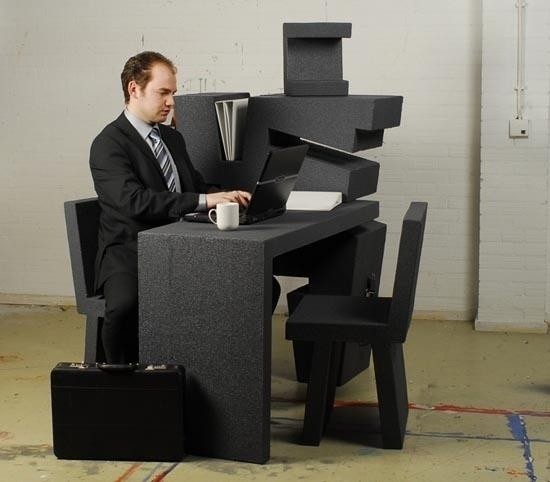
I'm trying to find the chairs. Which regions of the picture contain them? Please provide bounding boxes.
[63,197,110,360]
[284,202,430,450]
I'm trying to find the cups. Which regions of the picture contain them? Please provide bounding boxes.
[208,202,240,231]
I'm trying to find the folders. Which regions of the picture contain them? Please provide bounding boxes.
[214,98,248,162]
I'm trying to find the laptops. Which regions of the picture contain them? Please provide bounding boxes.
[183,144,309,225]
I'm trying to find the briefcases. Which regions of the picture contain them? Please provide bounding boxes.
[50,361,188,463]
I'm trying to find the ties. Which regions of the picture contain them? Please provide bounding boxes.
[148,127,177,193]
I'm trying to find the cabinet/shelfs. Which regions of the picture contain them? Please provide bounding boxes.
[169,22,405,200]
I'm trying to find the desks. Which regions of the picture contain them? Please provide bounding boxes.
[139,201,406,466]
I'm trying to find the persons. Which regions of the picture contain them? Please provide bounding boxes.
[85,48,253,366]
